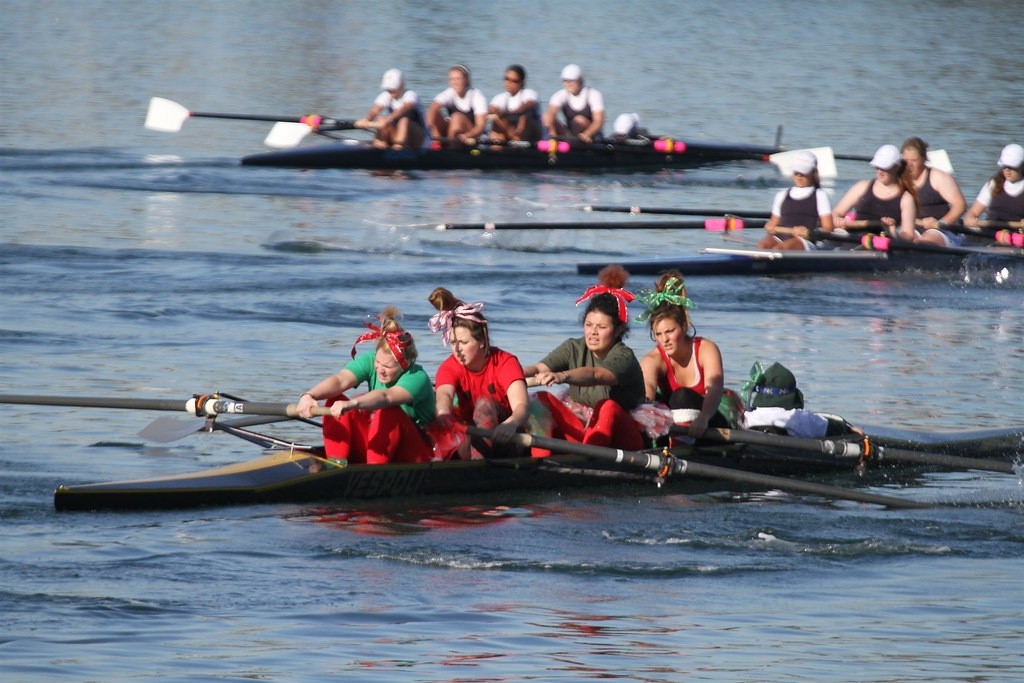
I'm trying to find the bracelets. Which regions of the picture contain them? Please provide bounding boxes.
[937,219,945,227]
[560,371,568,383]
[299,392,314,398]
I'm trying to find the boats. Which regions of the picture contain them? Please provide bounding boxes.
[576,246,1024,274]
[241,142,780,171]
[53,423,1024,511]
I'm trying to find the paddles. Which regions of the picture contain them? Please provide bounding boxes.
[957,219,1023,231]
[915,218,1024,248]
[513,195,857,221]
[430,135,571,157]
[526,377,550,387]
[434,214,894,233]
[264,120,359,149]
[141,94,383,139]
[0,393,345,419]
[752,144,954,176]
[763,224,1024,265]
[137,415,290,444]
[444,422,923,509]
[667,425,1019,474]
[557,137,688,163]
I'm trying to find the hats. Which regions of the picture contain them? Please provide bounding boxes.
[740,361,804,411]
[870,145,903,170]
[997,144,1024,167]
[791,150,817,175]
[614,113,640,135]
[379,69,404,89]
[560,64,581,81]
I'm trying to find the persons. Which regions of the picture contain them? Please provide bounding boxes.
[486,64,543,149]
[757,151,834,250]
[965,143,1024,245]
[428,288,530,460]
[635,270,735,449]
[719,362,866,438]
[296,306,436,465]
[427,65,488,149]
[540,65,605,143]
[831,139,968,249]
[524,265,652,459]
[355,69,426,147]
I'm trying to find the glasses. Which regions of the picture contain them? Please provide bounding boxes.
[504,75,522,83]
